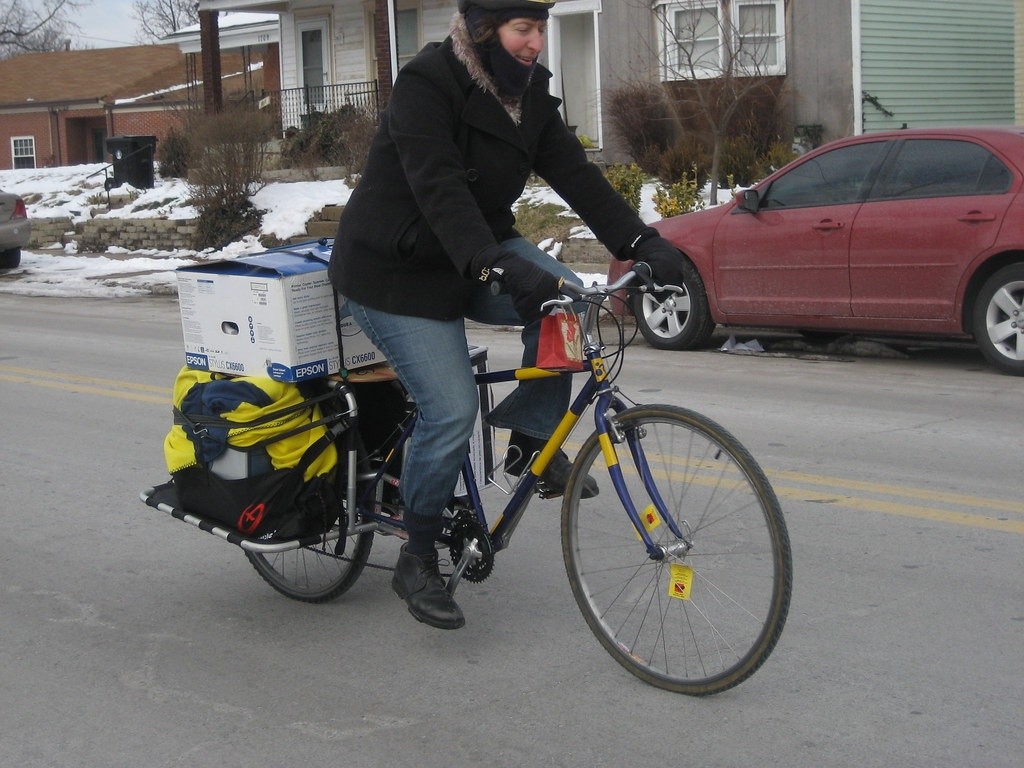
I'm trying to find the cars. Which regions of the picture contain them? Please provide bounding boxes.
[0,189,32,267]
[607,125,1023,375]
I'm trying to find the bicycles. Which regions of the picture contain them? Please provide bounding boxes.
[139,260,797,696]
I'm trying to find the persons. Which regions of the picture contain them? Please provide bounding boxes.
[326,0,683,629]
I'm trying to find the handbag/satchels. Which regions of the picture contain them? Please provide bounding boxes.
[536,296,584,372]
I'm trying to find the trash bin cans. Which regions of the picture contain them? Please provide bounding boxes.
[105,135,156,192]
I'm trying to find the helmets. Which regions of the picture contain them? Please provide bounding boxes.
[457,0,556,14]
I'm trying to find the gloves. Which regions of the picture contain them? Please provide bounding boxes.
[624,227,684,303]
[471,243,558,322]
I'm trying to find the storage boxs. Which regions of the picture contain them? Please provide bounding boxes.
[174,235,387,383]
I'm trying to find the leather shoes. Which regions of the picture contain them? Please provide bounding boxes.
[392,542,465,629]
[504,430,599,499]
